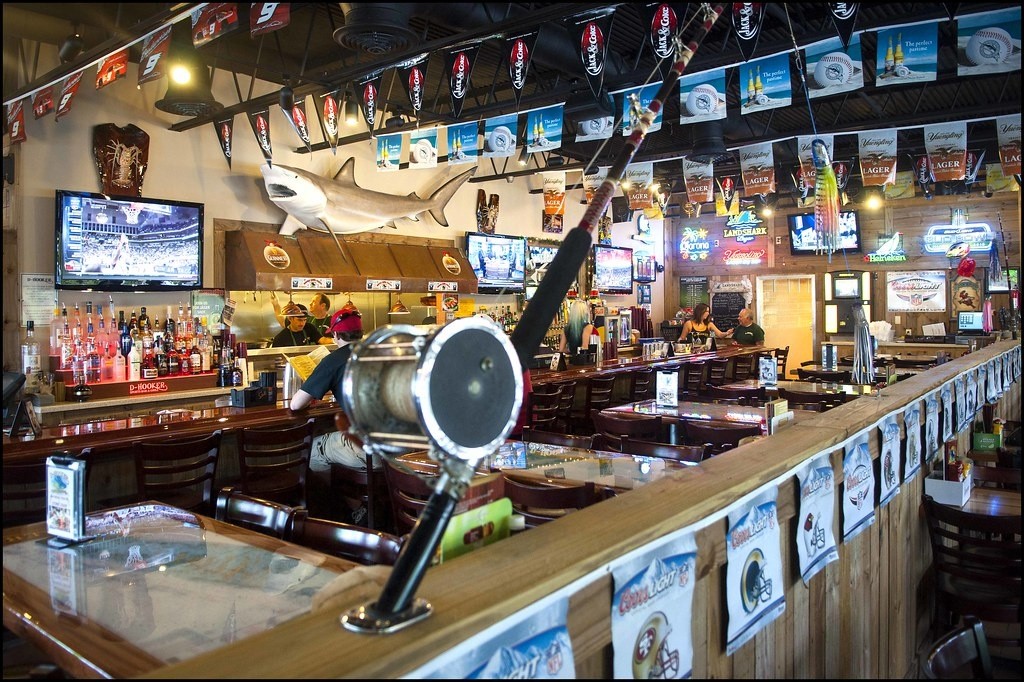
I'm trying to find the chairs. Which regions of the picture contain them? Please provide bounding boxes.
[0,345,1024,682]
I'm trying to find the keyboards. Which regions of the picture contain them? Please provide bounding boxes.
[963,332,983,336]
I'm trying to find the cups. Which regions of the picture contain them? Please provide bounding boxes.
[642,341,665,361]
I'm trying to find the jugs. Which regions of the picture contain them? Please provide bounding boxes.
[282,362,302,400]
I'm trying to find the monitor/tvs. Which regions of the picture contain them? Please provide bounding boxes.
[593,242,634,295]
[464,232,525,295]
[787,209,862,256]
[54,190,203,293]
[957,310,984,333]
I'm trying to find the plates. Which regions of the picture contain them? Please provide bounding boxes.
[442,297,458,311]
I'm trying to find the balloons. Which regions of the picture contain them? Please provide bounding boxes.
[958,257,975,278]
[946,242,970,259]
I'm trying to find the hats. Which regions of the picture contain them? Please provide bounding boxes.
[291,304,312,318]
[325,308,363,334]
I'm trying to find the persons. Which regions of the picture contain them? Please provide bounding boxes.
[560,300,594,354]
[270,292,333,338]
[290,309,414,527]
[272,304,335,347]
[731,309,764,346]
[676,303,734,351]
[111,234,130,275]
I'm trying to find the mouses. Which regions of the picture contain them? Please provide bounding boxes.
[984,333,990,336]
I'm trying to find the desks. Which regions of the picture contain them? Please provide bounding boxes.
[968,444,1024,463]
[949,488,1024,566]
[720,380,875,404]
[846,354,937,365]
[599,398,815,437]
[789,366,924,380]
[400,439,701,504]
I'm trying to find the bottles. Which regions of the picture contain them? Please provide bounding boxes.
[472,297,571,350]
[709,330,715,338]
[50,300,243,388]
[20,320,44,392]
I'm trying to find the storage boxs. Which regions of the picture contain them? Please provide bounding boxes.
[925,470,970,508]
[972,431,1002,451]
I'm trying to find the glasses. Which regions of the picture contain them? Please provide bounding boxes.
[331,310,362,330]
